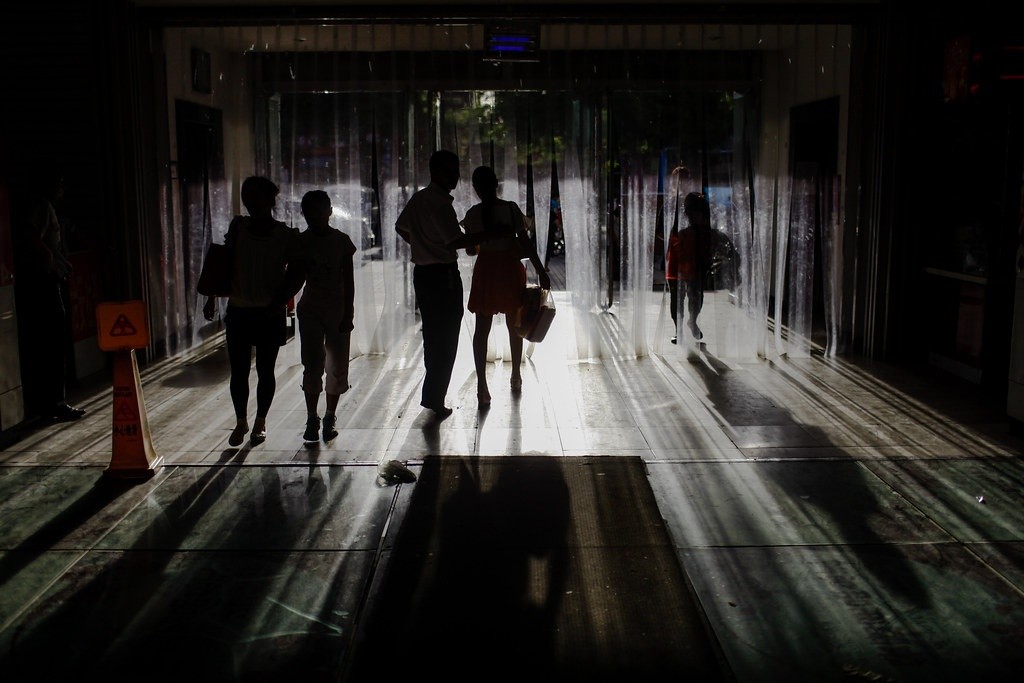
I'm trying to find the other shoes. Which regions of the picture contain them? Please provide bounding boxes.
[510,374,522,391]
[421,398,452,415]
[477,389,491,405]
[303,417,321,441]
[229,423,249,446]
[687,321,703,339]
[671,334,682,344]
[250,420,266,442]
[322,416,338,442]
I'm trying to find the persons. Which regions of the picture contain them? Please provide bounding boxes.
[289,187,355,441]
[203,176,298,446]
[666,165,706,343]
[458,165,552,400]
[24,168,86,422]
[395,149,471,415]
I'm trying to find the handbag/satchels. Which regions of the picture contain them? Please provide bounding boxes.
[197,243,230,297]
[516,286,555,342]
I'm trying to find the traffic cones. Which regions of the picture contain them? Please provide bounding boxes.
[93,298,166,479]
